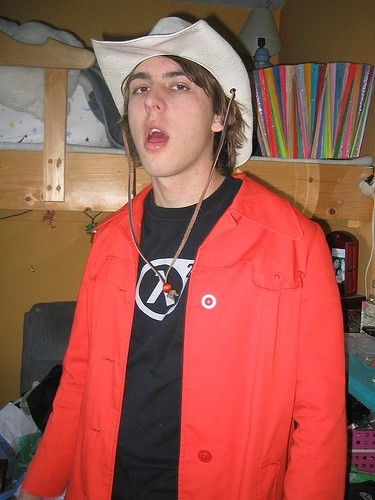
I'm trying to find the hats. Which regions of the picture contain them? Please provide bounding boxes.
[90,16,254,168]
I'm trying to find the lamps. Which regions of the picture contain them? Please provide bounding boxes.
[234,7,282,70]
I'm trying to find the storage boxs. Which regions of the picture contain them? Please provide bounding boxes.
[348,428,375,472]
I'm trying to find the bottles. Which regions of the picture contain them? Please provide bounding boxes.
[254,47,273,70]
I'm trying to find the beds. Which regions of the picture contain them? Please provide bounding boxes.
[1,31,374,229]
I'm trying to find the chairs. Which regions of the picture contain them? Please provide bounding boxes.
[0,299,78,493]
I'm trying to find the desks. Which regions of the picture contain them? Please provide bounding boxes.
[345,351,374,415]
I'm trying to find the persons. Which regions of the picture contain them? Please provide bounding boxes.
[19,14,345,500]
